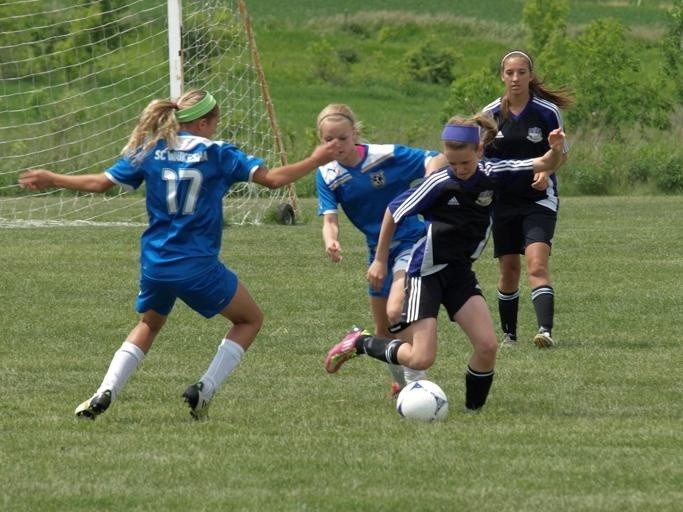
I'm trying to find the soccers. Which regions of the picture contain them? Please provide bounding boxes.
[396,379,448,422]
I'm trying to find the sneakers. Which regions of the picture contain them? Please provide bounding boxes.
[532,326,555,349]
[182,381,212,423]
[387,382,401,405]
[74,390,111,421]
[323,324,373,373]
[499,332,518,349]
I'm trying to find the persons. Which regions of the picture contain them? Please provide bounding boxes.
[16,86,342,420]
[322,110,569,418]
[313,101,450,400]
[472,50,576,351]
